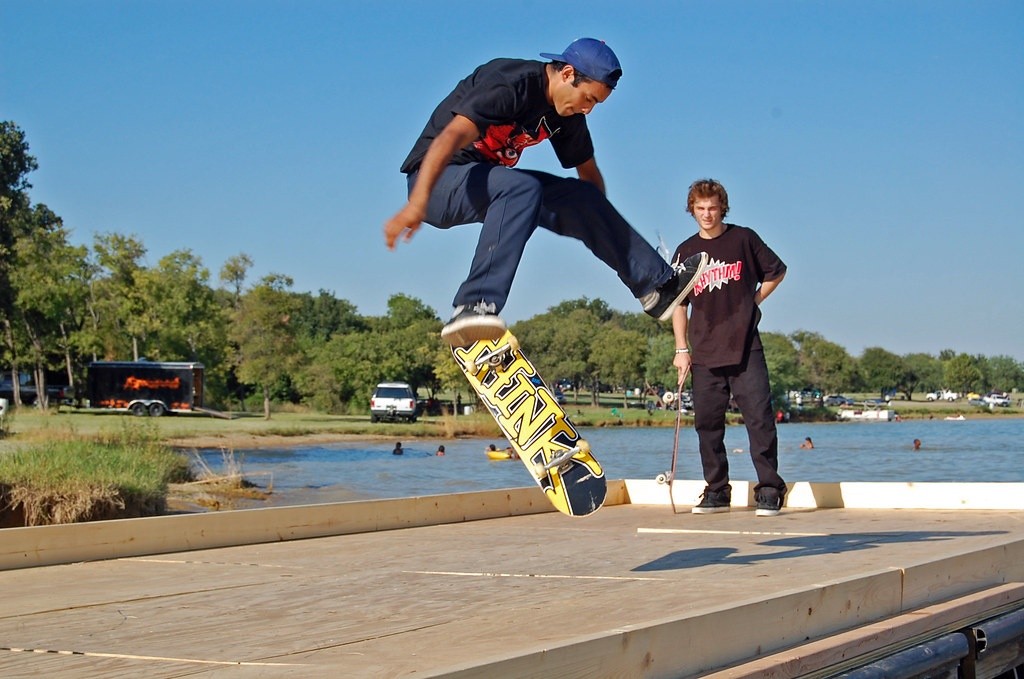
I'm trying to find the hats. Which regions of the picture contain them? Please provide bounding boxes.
[540,38,623,90]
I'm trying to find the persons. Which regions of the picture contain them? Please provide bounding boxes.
[436,445,445,456]
[669,180,787,516]
[912,439,921,450]
[800,437,813,449]
[393,442,403,454]
[386,37,706,347]
[485,444,520,460]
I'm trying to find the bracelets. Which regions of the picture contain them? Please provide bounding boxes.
[675,349,690,353]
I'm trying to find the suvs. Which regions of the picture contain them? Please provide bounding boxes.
[370,381,417,423]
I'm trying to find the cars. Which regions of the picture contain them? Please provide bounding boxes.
[555,390,566,403]
[826,396,853,407]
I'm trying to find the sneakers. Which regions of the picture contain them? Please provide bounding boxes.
[645,252,709,321]
[439,307,506,345]
[690,492,731,514]
[755,486,783,516]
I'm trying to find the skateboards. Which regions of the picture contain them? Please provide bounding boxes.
[449,327,607,516]
[655,360,690,514]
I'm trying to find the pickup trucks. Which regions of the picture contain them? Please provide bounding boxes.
[983,394,1009,406]
[927,390,958,402]
[0,381,63,406]
